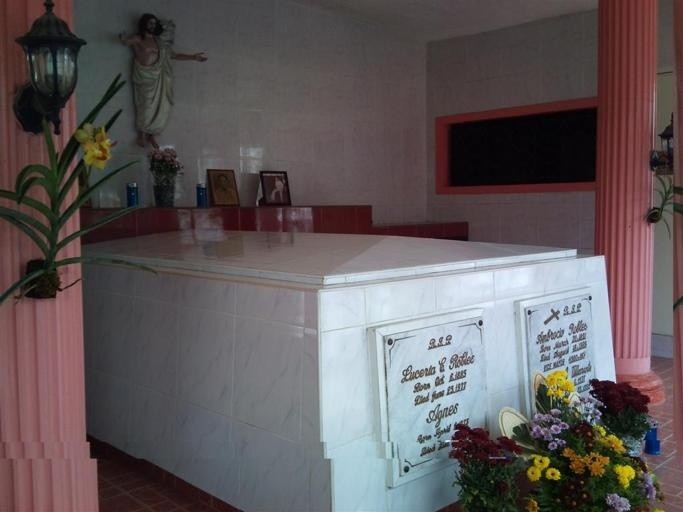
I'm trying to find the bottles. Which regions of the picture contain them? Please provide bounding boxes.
[196,183,208,208]
[126,182,138,208]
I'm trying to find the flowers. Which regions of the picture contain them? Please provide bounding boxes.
[447,369,668,512]
[0,71,163,298]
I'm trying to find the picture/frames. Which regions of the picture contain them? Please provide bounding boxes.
[206,169,240,207]
[255,170,292,207]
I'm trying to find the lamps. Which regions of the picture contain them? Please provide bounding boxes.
[13,1,88,136]
[649,112,675,175]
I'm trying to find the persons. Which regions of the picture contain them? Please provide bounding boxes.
[120,14,208,149]
[216,174,237,202]
[271,177,284,203]
